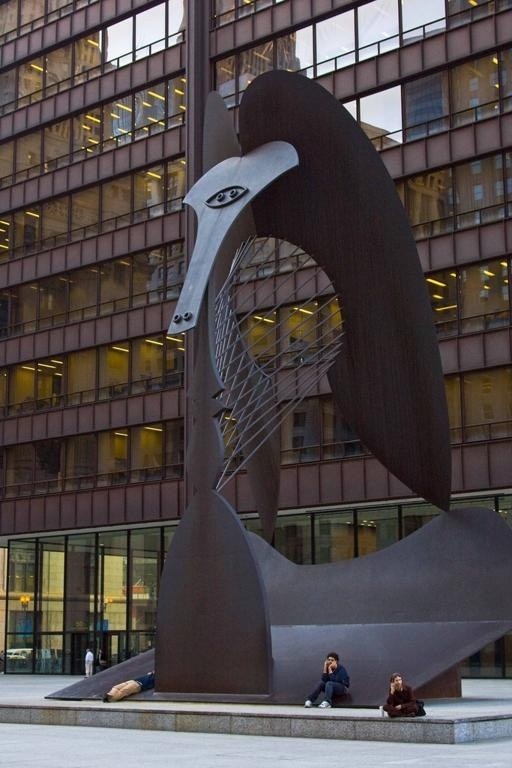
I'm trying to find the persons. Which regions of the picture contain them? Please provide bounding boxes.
[305,652,351,708]
[103,671,155,703]
[96,647,106,672]
[383,673,418,719]
[84,648,95,679]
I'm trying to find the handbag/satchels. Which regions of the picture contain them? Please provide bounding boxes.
[417,699,426,716]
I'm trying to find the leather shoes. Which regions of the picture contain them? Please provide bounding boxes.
[103,690,108,703]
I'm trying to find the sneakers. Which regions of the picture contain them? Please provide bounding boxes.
[304,700,313,708]
[318,701,332,708]
